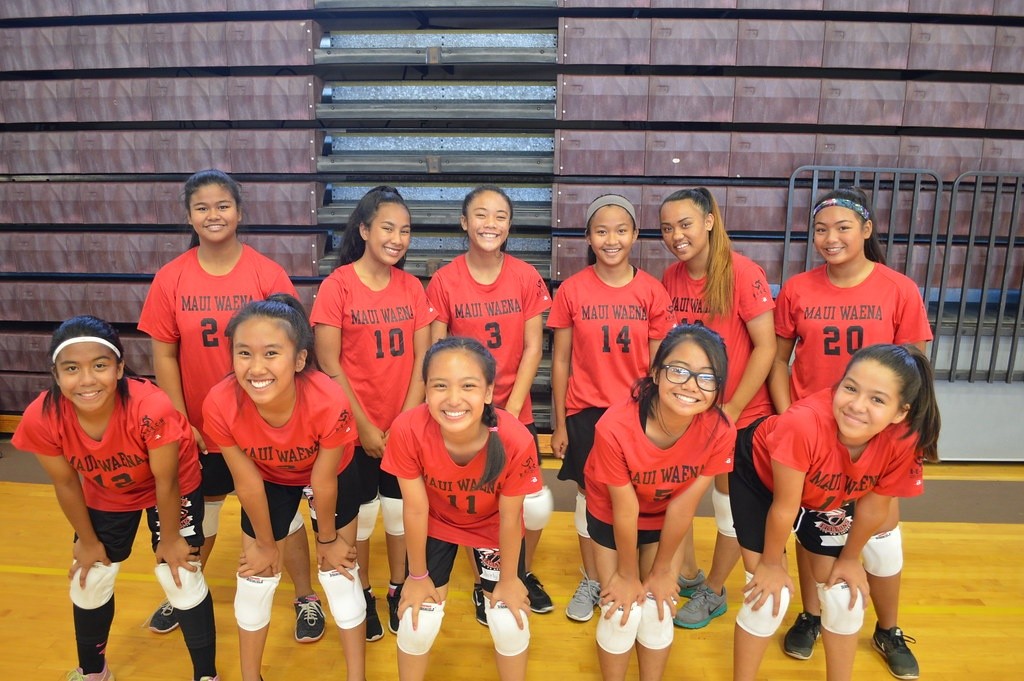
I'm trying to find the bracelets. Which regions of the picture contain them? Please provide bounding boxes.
[407,570,429,579]
[315,533,337,544]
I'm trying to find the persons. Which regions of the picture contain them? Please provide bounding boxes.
[379,336,544,681]
[546,193,678,623]
[726,344,942,681]
[309,184,438,643]
[422,185,555,627]
[767,188,936,680]
[657,185,778,629]
[11,314,218,681]
[136,170,326,642]
[203,293,366,681]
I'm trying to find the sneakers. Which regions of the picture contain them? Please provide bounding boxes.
[293,591,326,642]
[565,565,601,622]
[674,585,728,628]
[65,661,114,681]
[387,586,407,634]
[520,572,554,613]
[473,583,490,627]
[143,597,179,634]
[194,673,220,681]
[677,569,708,598]
[364,591,383,641]
[783,612,821,659]
[873,621,919,679]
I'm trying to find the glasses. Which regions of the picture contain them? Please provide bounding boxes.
[659,365,722,392]
